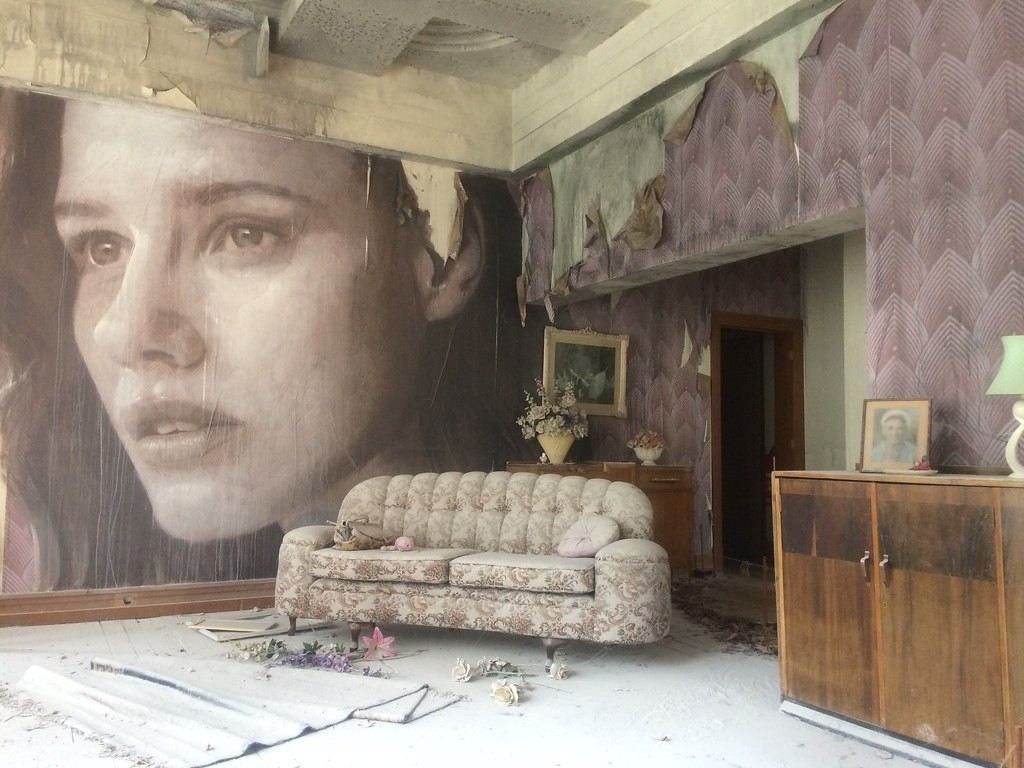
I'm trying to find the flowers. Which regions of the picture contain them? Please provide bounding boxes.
[626,430,666,449]
[363,628,571,708]
[516,377,588,438]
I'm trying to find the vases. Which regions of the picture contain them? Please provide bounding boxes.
[634,447,663,466]
[537,428,575,465]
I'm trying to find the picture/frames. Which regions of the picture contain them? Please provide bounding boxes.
[543,327,629,419]
[859,398,933,475]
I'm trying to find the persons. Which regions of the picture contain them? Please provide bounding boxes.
[872,409,916,460]
[0,91,523,589]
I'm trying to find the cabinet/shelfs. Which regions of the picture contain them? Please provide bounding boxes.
[771,470,1024,768]
[509,459,695,584]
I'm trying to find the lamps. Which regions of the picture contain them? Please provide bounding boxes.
[988,335,1024,478]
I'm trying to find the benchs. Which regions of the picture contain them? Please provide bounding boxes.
[273,472,672,672]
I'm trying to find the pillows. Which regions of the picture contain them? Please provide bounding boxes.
[558,514,619,558]
